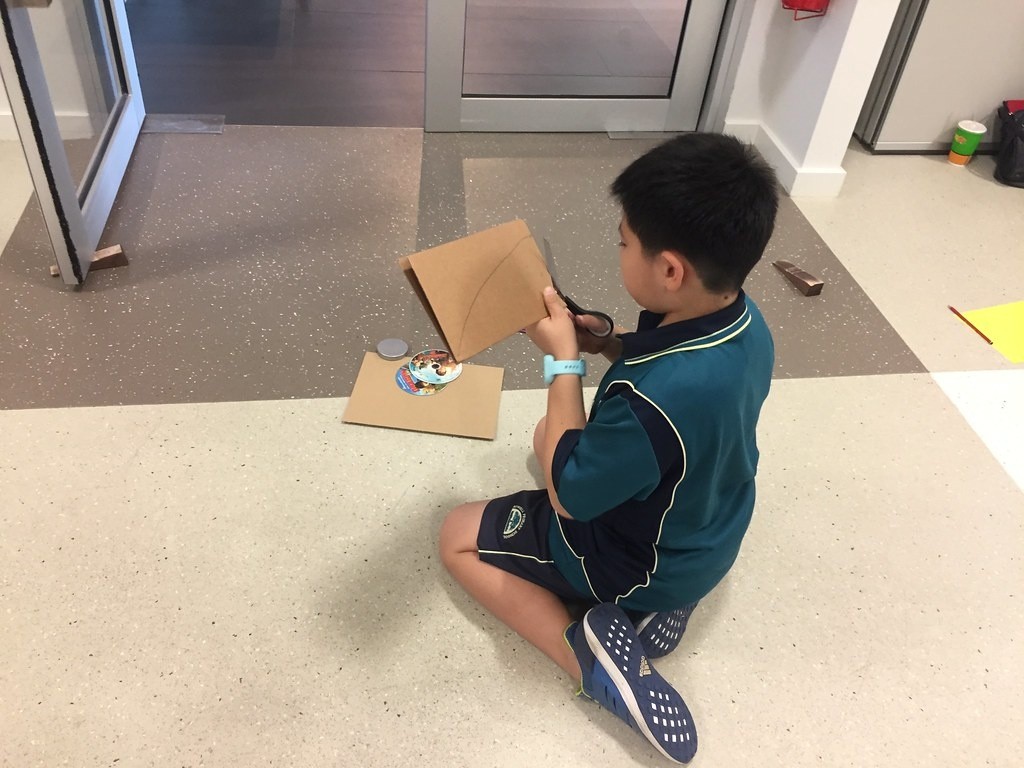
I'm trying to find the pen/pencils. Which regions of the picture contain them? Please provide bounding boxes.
[947,304,993,344]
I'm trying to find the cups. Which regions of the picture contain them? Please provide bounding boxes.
[947,120,987,166]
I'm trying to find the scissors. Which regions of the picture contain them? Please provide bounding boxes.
[540,237,613,339]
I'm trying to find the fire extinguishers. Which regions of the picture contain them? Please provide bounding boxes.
[781,0,826,13]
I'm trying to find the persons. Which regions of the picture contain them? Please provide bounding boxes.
[437,131,776,763]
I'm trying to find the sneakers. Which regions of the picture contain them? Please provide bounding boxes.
[633,598,700,660]
[563,602,697,764]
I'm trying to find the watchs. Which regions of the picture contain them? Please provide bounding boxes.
[541,352,586,385]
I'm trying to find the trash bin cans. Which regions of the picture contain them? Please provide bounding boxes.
[994,100,1024,188]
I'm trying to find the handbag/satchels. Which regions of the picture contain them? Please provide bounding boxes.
[993,100,1024,189]
